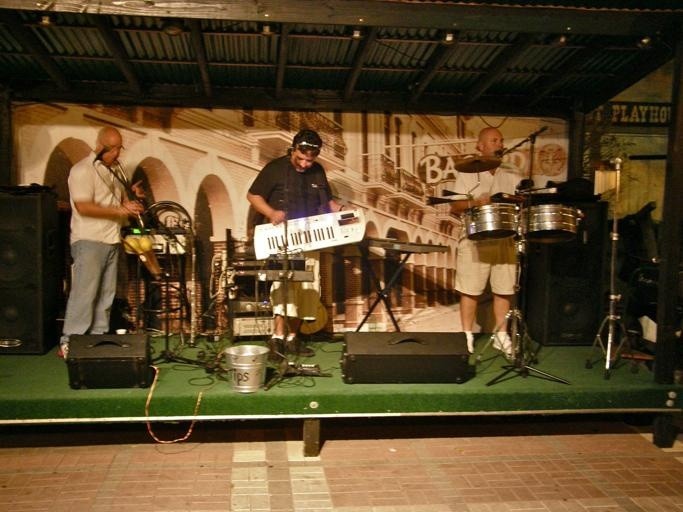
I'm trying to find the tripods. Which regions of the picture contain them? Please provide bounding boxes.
[263,162,332,391]
[99,160,208,368]
[474,236,539,365]
[485,125,572,386]
[586,157,639,380]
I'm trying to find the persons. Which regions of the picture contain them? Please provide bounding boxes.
[57,124,148,362]
[448,126,534,364]
[246,128,359,365]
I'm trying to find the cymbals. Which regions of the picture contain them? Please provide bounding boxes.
[454,155,501,172]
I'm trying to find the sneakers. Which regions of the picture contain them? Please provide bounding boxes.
[492,336,512,356]
[60,343,69,359]
[270,338,284,363]
[284,337,315,357]
[467,335,474,353]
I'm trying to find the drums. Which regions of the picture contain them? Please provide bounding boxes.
[517,203,584,243]
[460,202,519,240]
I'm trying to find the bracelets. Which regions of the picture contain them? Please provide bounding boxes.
[339,204,345,212]
[466,199,473,209]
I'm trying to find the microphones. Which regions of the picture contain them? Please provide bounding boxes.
[94,144,110,162]
[496,148,503,155]
[286,149,292,162]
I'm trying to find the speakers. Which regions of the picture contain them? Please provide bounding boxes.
[518,201,608,346]
[340,332,470,384]
[66,334,154,389]
[0,192,59,355]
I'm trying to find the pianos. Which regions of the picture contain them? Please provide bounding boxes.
[351,236,449,253]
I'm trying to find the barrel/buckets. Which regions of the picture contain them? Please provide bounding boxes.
[223,345,271,393]
